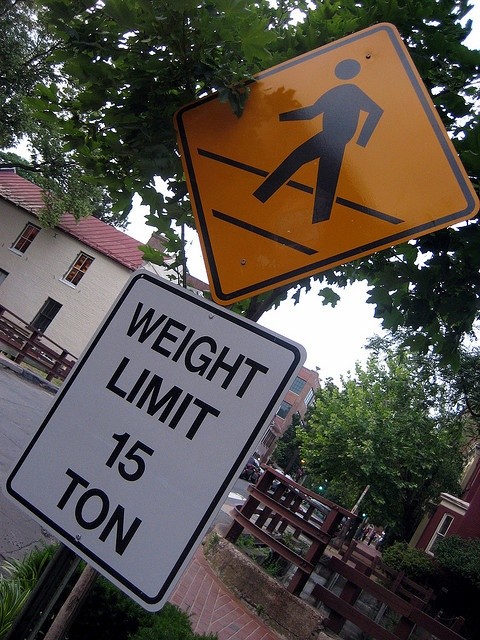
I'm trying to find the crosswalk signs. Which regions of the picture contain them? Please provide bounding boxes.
[169,22,478,306]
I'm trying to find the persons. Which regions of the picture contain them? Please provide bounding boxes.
[361,527,370,542]
[367,531,376,546]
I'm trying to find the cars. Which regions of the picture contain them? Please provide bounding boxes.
[240,455,301,501]
